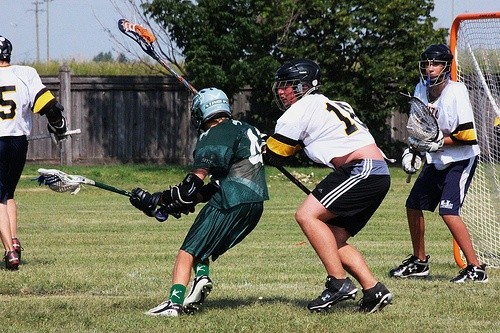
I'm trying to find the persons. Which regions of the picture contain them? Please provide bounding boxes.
[389,44,489,284]
[261,58,394,315]
[0,35,67,271]
[130,88,270,316]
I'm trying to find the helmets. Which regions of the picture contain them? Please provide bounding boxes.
[417,44,453,88]
[0,35,12,63]
[275,58,320,94]
[190,87,232,125]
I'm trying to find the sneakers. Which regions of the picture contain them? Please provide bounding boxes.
[3,250,19,271]
[450,263,489,284]
[142,300,183,317]
[12,238,24,259]
[387,253,431,280]
[183,275,213,316]
[307,275,358,314]
[356,281,392,315]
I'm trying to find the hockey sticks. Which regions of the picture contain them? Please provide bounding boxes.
[27,128,82,142]
[37,167,182,220]
[117,17,312,195]
[406,95,440,184]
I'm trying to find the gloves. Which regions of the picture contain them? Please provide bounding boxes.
[129,184,195,222]
[47,116,72,145]
[408,129,445,153]
[401,146,422,175]
